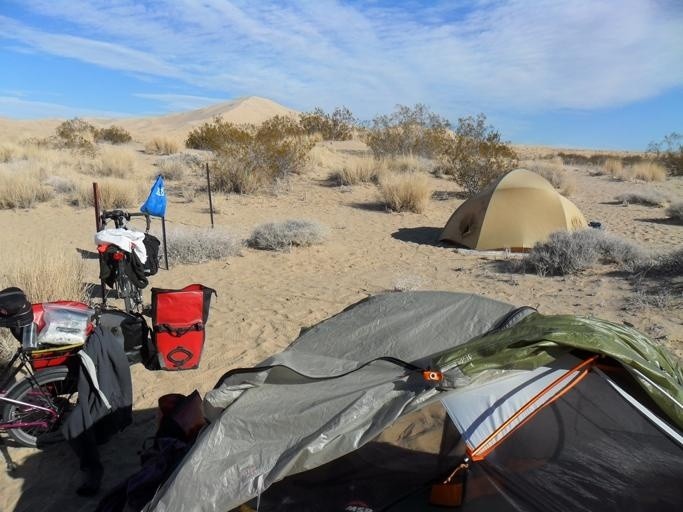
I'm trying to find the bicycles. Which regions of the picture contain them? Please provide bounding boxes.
[0,286,95,449]
[98,211,150,313]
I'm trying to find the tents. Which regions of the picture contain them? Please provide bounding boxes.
[437,168,587,251]
[137,290,681,512]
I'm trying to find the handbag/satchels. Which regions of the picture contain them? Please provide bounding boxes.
[103,310,149,364]
[141,233,161,276]
[140,176,167,218]
